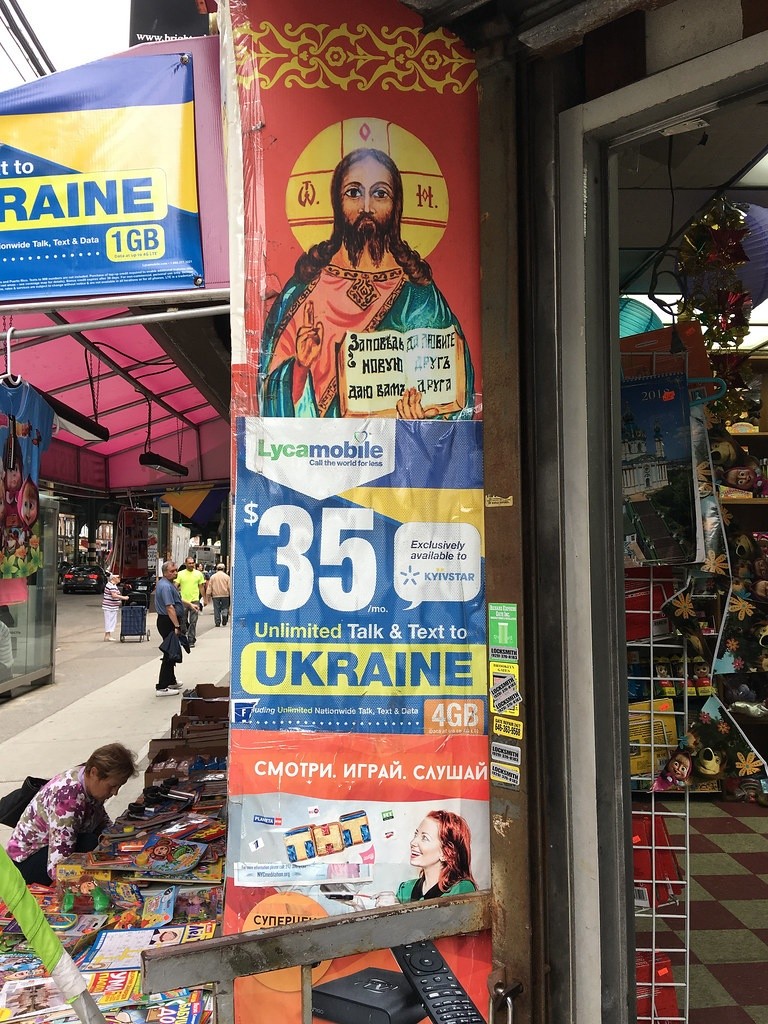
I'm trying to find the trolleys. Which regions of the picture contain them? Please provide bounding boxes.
[120,605,153,644]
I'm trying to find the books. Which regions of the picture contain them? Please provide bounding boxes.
[0,683,230,1023]
[627,698,678,775]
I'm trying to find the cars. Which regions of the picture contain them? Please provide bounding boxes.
[62,563,111,594]
[57,560,71,586]
[121,575,156,610]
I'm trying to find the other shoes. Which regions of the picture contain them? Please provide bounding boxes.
[189,644,195,648]
[216,623,220,626]
[103,637,108,642]
[223,621,226,625]
[108,637,117,642]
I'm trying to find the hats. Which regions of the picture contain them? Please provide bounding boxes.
[217,563,225,569]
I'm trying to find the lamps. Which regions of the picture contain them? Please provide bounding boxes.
[139,397,188,477]
[1,316,109,443]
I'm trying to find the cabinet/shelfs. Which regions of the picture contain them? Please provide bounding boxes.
[701,346,767,790]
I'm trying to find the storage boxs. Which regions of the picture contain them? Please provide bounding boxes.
[635,945,680,1024]
[145,683,230,787]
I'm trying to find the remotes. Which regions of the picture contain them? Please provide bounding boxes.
[390,939,487,1024]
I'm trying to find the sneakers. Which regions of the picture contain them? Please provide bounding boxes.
[156,688,180,696]
[168,681,183,688]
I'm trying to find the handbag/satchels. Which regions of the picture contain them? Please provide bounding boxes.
[0,776,49,828]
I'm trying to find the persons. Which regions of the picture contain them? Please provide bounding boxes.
[7,742,136,887]
[344,810,478,912]
[102,572,130,642]
[173,556,229,648]
[0,620,13,681]
[155,561,199,696]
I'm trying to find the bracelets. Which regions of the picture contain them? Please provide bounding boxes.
[174,625,181,629]
